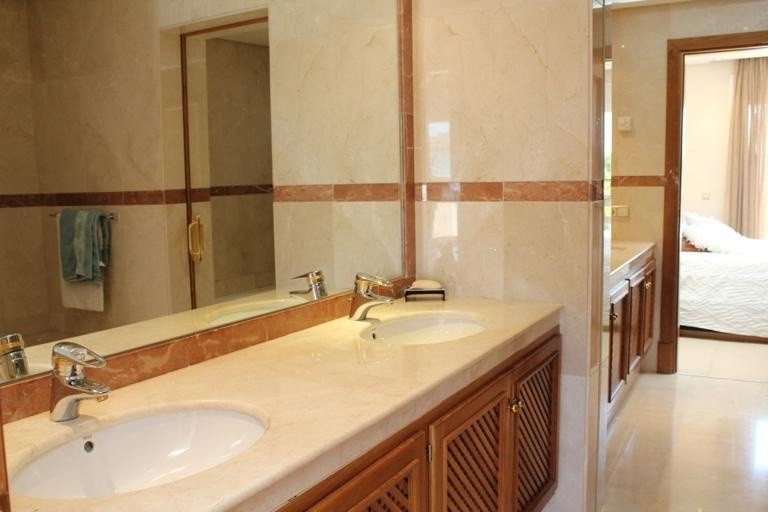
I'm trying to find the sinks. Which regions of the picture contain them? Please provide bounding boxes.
[610,244,626,257]
[8,401,270,500]
[206,299,307,327]
[358,312,492,347]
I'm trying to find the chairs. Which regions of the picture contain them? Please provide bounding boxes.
[607,248,656,427]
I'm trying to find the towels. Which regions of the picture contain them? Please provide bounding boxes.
[55,208,111,312]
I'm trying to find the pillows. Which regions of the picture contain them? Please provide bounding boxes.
[682,212,745,253]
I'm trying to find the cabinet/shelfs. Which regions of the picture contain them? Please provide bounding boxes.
[275,324,562,512]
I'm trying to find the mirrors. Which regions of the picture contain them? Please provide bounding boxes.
[0,2,417,425]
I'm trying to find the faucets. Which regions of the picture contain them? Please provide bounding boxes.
[289,270,327,301]
[0,333,29,383]
[49,342,112,422]
[348,273,398,320]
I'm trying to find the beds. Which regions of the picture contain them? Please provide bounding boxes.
[678,235,768,344]
